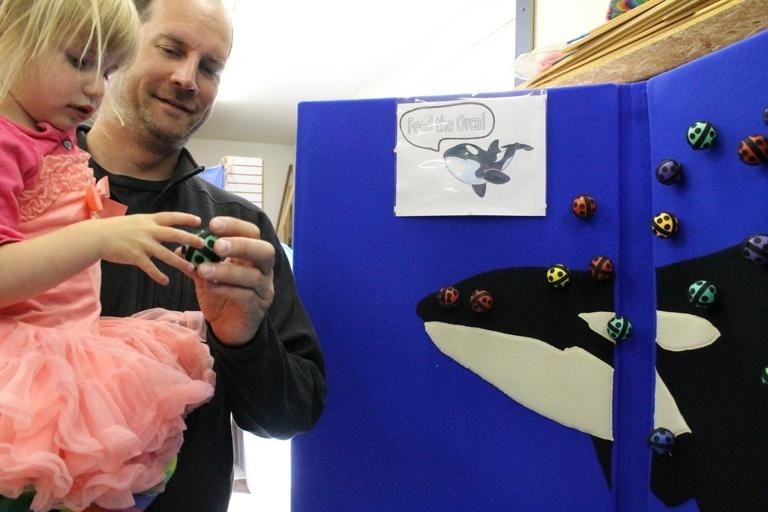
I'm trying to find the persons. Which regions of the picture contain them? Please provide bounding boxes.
[73,0,327,512]
[0,0,207,512]
